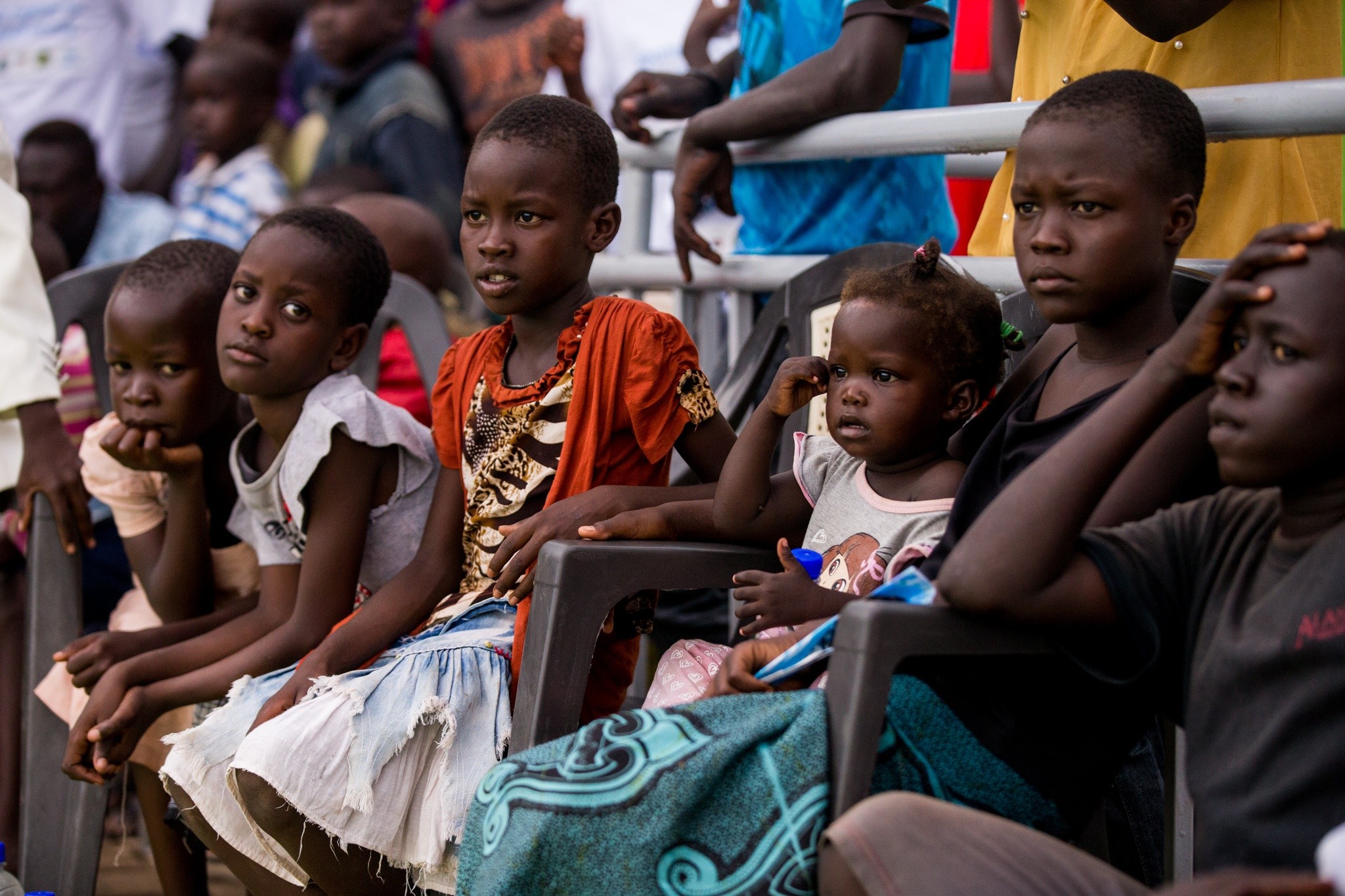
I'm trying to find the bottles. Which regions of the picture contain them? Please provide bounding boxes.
[788,549,823,587]
[0,841,25,896]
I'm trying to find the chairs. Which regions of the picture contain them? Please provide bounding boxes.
[20,243,1219,896]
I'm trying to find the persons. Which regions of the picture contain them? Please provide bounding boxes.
[0,0,1344,896]
[159,94,737,896]
